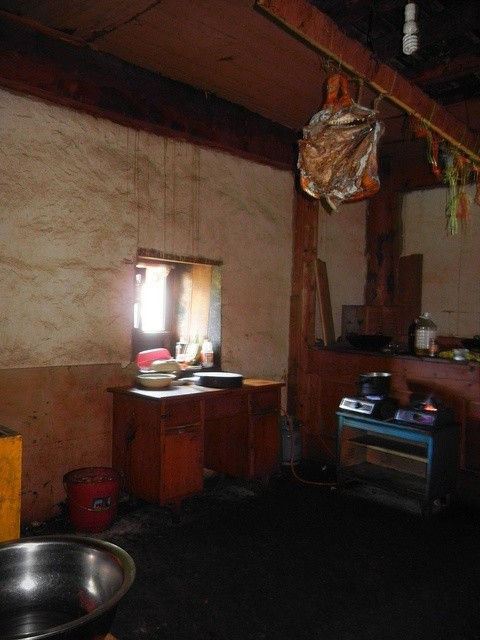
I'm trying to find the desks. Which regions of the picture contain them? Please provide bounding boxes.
[336,412,459,515]
[106,376,286,524]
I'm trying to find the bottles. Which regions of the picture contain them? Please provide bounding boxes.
[428,338,440,357]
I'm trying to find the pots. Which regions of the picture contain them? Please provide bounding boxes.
[179,371,244,388]
[359,370,394,397]
[345,334,394,353]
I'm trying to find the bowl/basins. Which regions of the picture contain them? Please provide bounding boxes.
[0,533,137,640]
[150,359,181,372]
[136,372,176,389]
[137,347,173,369]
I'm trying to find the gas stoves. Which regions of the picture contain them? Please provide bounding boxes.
[338,394,400,418]
[393,400,451,428]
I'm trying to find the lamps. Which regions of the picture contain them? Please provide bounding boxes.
[402,0,419,55]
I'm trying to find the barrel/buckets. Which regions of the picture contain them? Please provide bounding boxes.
[410,311,436,356]
